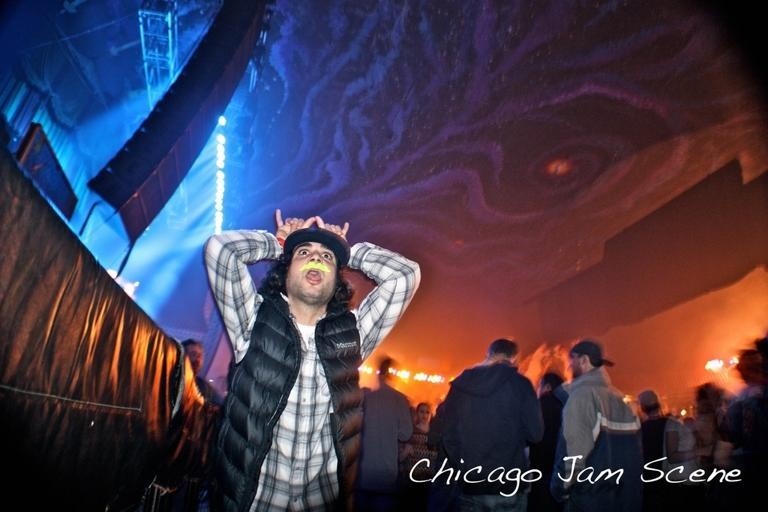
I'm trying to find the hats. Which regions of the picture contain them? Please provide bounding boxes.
[284,228,350,268]
[572,342,614,366]
[639,390,658,406]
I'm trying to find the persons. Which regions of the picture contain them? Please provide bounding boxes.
[203,210,423,511]
[180,338,765,509]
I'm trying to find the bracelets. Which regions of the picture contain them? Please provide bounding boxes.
[276,235,287,250]
